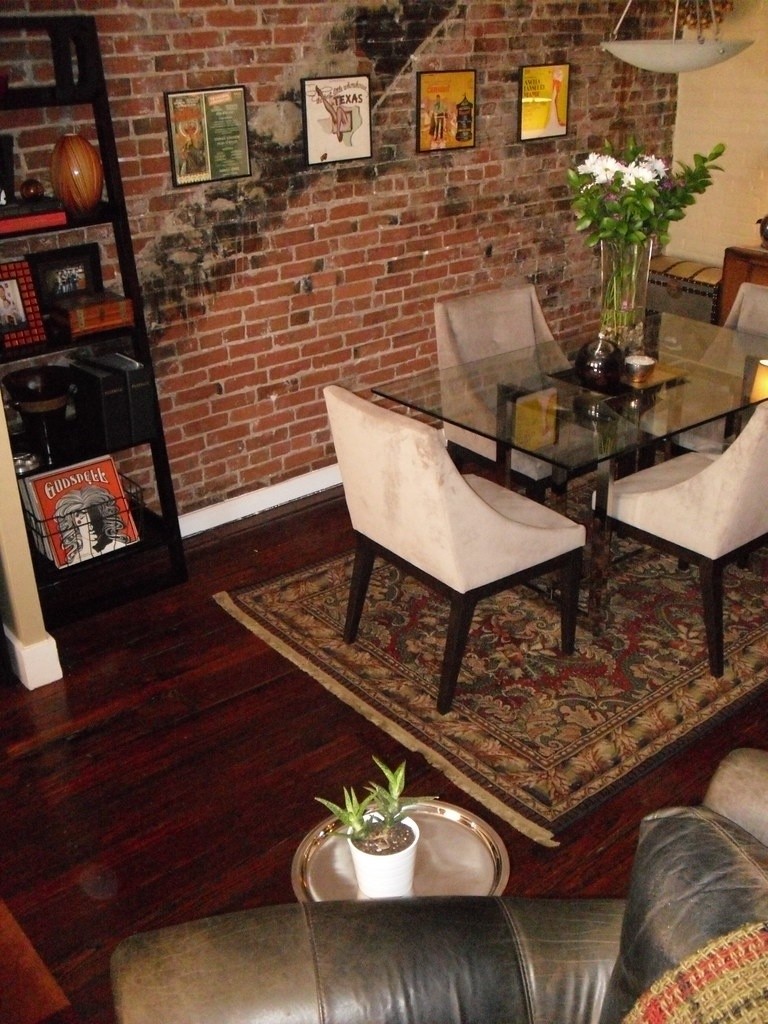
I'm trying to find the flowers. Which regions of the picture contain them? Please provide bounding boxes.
[566,134,725,249]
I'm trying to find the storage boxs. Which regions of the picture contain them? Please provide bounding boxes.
[49,288,135,338]
[646,256,723,324]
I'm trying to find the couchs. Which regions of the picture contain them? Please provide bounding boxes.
[110,747,767,1024]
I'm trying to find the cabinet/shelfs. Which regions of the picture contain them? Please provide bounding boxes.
[0,15,190,629]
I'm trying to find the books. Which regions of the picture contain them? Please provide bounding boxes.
[17,454,140,570]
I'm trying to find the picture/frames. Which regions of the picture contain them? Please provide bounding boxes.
[162,86,252,187]
[300,75,373,167]
[416,69,476,153]
[25,243,104,315]
[0,258,49,349]
[518,64,570,142]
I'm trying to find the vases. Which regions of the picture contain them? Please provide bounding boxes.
[600,238,653,355]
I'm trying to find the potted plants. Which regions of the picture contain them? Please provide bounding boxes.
[312,754,440,900]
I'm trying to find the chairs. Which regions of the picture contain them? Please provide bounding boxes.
[432,284,637,540]
[590,400,767,679]
[322,385,586,715]
[638,282,767,572]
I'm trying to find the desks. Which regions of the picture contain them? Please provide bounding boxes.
[370,311,768,636]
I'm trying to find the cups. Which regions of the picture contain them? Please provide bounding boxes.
[2,365,76,444]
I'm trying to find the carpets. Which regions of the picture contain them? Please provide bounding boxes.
[212,455,768,848]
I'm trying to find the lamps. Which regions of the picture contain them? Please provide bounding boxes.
[598,0,756,73]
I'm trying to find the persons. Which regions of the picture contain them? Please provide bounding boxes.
[0,286,22,327]
[55,268,81,295]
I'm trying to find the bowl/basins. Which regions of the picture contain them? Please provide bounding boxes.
[625,356,656,382]
[13,453,40,475]
